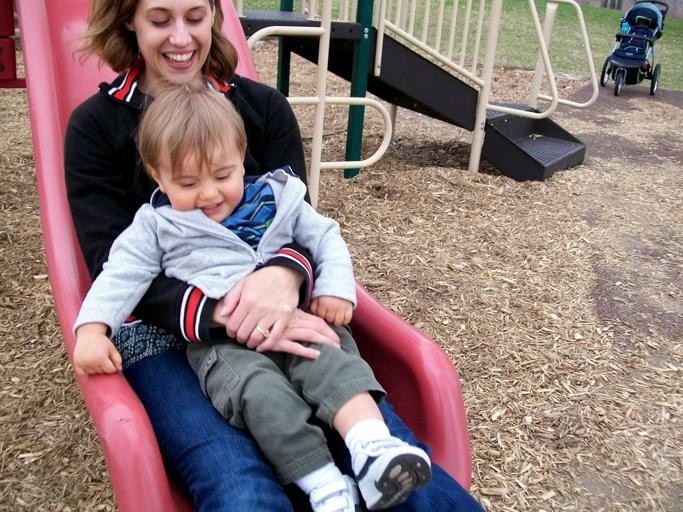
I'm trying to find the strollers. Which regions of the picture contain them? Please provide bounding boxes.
[600,0,669,97]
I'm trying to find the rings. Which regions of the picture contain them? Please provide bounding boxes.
[255,325,267,340]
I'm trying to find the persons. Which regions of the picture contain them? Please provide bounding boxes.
[64,81,433,509]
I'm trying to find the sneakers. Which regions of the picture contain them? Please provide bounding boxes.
[307,436,433,512]
